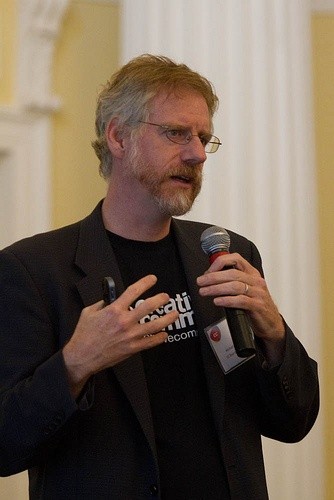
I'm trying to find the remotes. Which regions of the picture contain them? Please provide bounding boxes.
[102,277,115,307]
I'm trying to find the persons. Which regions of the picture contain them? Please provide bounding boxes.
[0,53,320,500]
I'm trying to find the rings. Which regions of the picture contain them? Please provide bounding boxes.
[243,283,249,294]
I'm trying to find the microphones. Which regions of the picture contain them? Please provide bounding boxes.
[201,226,257,358]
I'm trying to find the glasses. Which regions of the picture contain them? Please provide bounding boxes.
[137,121,222,153]
